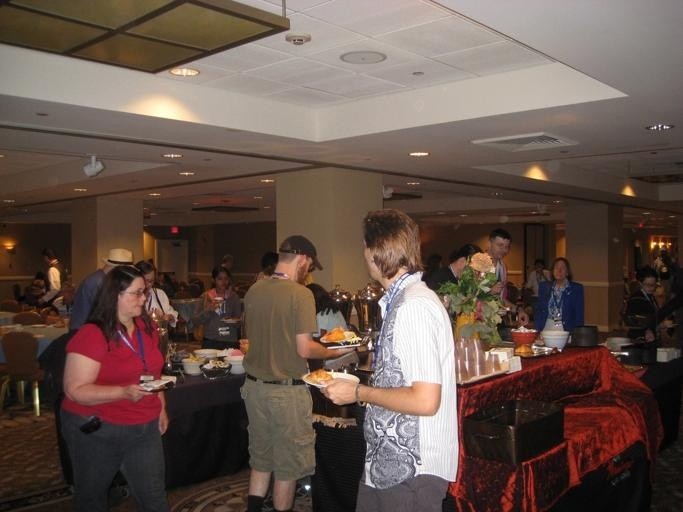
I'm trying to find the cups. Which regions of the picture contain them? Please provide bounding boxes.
[455,338,502,382]
[215,297,224,315]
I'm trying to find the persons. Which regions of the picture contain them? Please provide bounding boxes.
[36,252,348,349]
[423,227,585,343]
[239,236,354,512]
[624,266,661,344]
[59,265,169,512]
[319,208,459,512]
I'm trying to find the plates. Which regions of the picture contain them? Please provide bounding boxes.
[301,371,360,391]
[220,318,240,323]
[319,336,351,343]
[135,382,176,392]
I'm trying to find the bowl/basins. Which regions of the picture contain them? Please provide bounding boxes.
[513,330,539,345]
[541,330,570,350]
[167,348,246,378]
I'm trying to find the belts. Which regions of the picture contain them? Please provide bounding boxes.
[246,374,305,386]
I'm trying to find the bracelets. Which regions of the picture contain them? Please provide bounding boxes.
[356,384,366,407]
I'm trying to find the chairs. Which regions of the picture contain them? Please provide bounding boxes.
[0,250,682,512]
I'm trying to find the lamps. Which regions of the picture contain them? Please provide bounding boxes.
[0,0,290,78]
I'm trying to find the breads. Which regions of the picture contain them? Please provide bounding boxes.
[306,369,332,382]
[324,328,345,341]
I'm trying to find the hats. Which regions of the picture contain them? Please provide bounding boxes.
[101,248,133,266]
[279,235,323,271]
